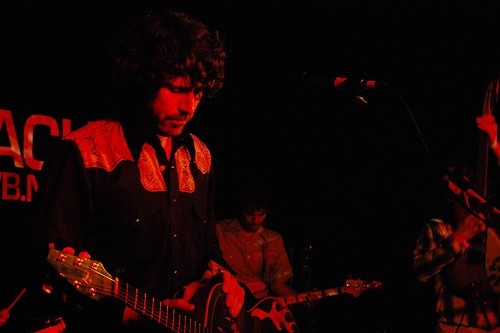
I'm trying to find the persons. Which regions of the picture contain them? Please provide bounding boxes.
[412,106,500,333]
[35,9,238,332]
[213,187,320,332]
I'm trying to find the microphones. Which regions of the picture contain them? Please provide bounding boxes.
[333,77,389,90]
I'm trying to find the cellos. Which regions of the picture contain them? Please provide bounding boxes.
[435,79,500,333]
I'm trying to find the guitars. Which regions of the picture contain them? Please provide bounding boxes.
[46,241,298,333]
[246,274,382,306]
